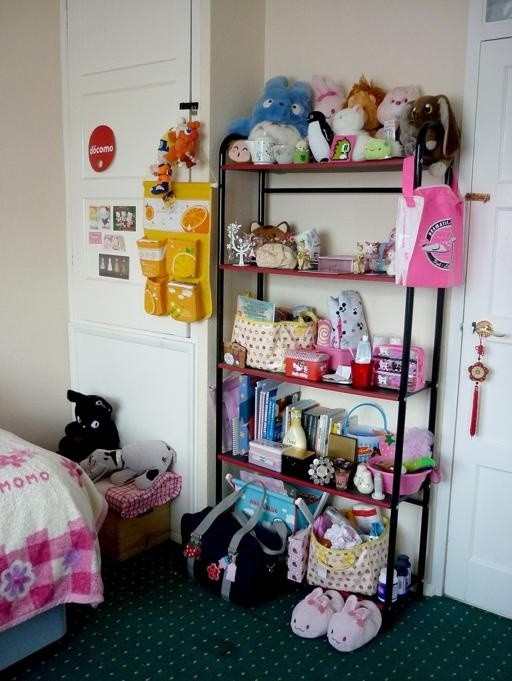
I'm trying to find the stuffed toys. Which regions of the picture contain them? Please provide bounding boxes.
[111,439,172,488]
[57,389,120,462]
[231,72,459,178]
[250,220,291,250]
[297,239,313,272]
[352,240,395,277]
[151,116,198,194]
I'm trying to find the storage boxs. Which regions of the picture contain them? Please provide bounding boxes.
[285,351,330,383]
[99,502,171,565]
[248,437,317,481]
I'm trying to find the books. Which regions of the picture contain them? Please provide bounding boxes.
[208,372,359,469]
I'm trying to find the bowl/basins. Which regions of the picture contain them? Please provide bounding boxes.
[367,455,433,496]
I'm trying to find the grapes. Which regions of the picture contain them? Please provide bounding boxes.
[185,541,199,559]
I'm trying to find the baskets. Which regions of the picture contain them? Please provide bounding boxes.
[343,402,387,462]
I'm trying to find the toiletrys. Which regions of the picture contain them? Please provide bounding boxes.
[282,408,307,449]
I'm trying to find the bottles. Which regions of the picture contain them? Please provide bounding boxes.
[356,334,371,362]
[322,506,367,544]
[378,555,413,602]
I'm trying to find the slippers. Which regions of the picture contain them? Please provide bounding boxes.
[291,587,345,638]
[326,593,383,651]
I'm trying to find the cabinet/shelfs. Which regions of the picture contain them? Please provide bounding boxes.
[214,121,454,628]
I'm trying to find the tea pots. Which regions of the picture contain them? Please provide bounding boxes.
[245,131,275,165]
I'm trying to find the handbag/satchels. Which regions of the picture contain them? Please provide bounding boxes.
[305,506,392,597]
[181,480,291,603]
[231,306,320,375]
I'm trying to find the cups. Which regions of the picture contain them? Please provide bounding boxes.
[274,144,293,165]
[350,361,369,387]
[334,458,352,490]
[295,227,321,269]
